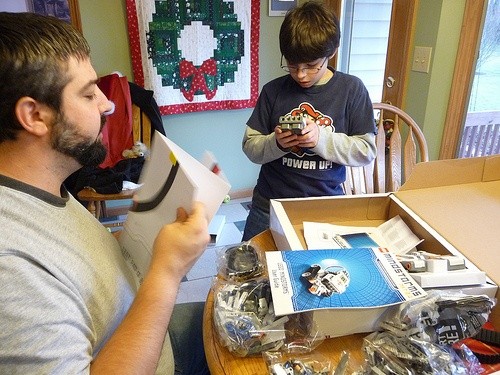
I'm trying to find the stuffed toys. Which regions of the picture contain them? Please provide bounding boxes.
[122,142,147,159]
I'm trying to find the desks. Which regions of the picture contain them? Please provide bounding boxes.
[202,229,371,375]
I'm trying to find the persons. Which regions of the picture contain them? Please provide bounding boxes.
[0,12,211,375]
[242,0,378,241]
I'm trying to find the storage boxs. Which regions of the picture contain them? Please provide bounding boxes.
[270,155,500,335]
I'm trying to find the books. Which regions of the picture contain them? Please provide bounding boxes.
[265,248,427,316]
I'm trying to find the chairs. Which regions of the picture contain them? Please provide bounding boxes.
[77,83,165,228]
[341,103,429,195]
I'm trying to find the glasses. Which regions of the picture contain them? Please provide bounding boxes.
[280,54,328,74]
